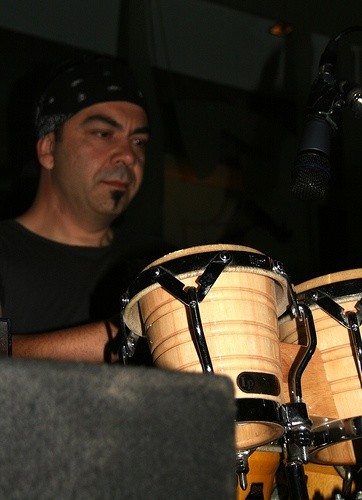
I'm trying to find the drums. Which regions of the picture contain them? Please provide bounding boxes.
[120,243,291,450]
[279,268,362,466]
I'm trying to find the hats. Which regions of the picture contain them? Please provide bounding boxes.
[34,64,150,139]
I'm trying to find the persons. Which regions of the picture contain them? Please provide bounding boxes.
[0,59,176,363]
